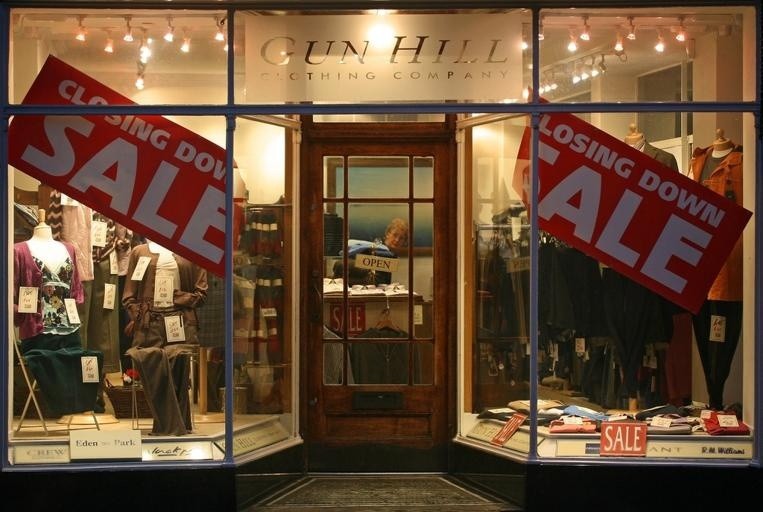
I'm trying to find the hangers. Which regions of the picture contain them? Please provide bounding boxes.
[373,297,401,334]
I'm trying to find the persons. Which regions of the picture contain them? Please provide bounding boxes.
[578,130,689,410]
[13,223,102,414]
[381,216,408,257]
[117,237,209,439]
[684,128,744,409]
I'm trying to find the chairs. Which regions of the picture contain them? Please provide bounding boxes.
[12,321,105,439]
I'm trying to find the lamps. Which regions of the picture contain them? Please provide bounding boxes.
[70,13,227,92]
[522,9,686,104]
